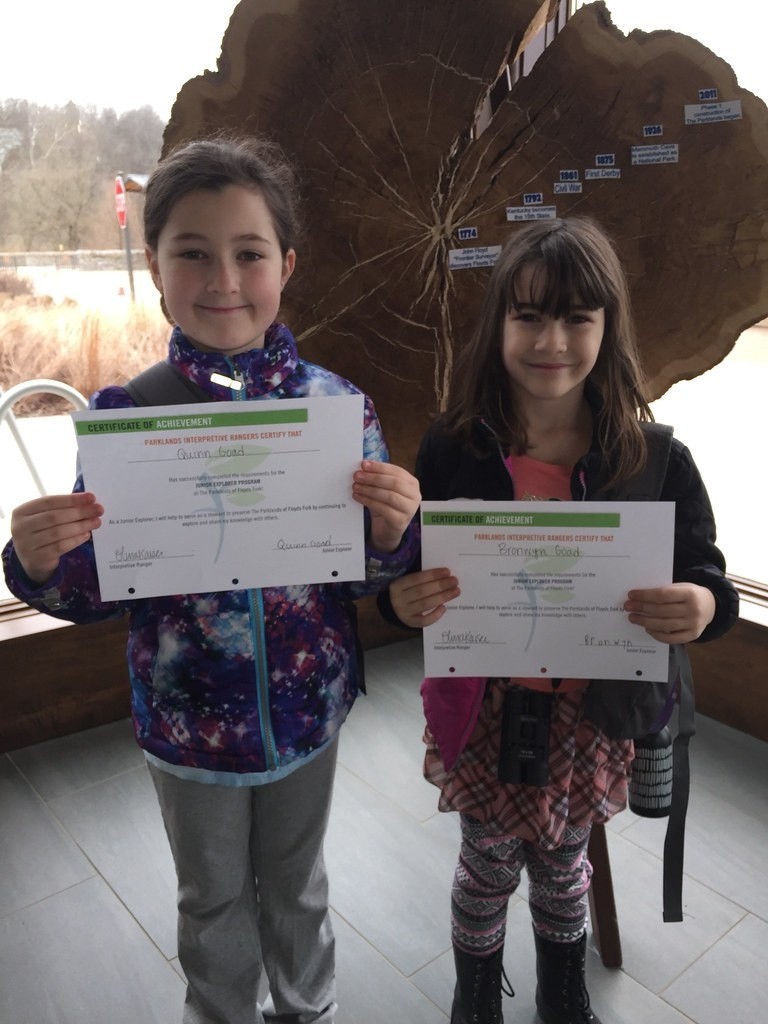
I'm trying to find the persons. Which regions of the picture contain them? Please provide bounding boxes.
[2,143,420,1024]
[375,220,740,1024]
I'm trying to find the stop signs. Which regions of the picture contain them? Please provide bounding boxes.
[114,176,127,229]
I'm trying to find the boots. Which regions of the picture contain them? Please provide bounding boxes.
[532,920,601,1024]
[450,942,514,1024]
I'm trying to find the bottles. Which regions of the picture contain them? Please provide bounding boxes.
[628,725,673,818]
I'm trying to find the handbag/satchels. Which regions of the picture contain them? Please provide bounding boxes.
[581,421,682,741]
